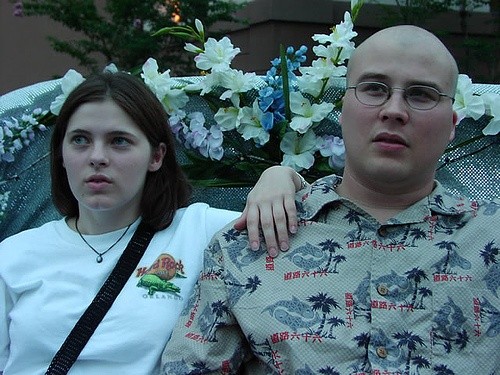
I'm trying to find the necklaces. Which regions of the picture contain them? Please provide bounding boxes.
[76,215,134,263]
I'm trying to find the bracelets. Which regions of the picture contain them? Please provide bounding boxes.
[296,171,307,192]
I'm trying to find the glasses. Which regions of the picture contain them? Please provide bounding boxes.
[344,82,456,111]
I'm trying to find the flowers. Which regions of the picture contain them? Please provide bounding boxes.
[1,0,499,219]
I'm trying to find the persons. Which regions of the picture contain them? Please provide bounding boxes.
[0,70,309,375]
[159,25,499,375]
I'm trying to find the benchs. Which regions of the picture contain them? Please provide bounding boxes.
[0,73,500,245]
